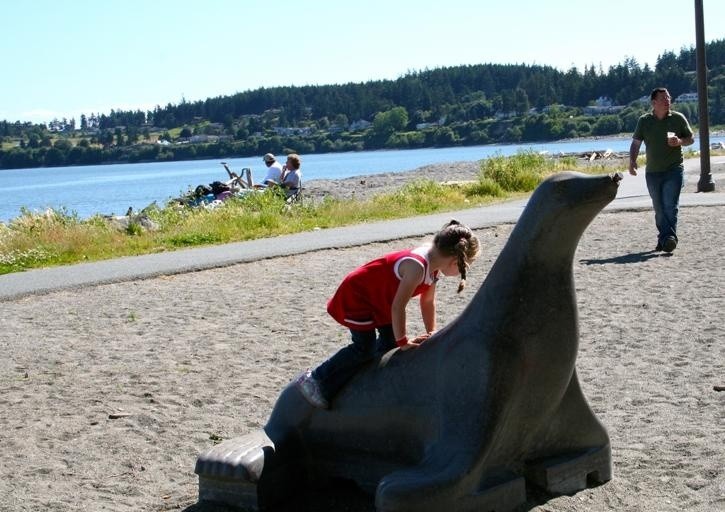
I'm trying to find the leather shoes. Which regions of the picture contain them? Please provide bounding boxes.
[656,234,677,253]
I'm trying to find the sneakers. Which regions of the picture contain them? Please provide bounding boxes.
[295,369,329,410]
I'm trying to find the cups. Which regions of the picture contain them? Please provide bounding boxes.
[667,132,675,138]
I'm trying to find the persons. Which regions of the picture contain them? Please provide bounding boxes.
[295,218,480,411]
[261,153,282,187]
[629,88,696,254]
[267,154,302,202]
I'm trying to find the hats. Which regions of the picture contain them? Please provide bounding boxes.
[263,153,275,162]
